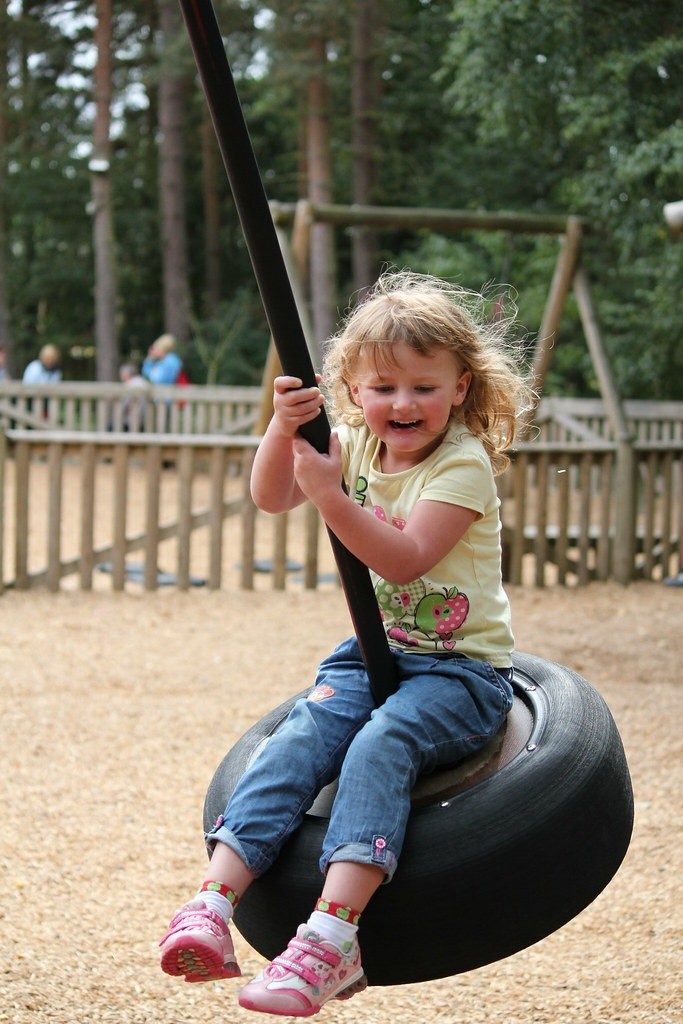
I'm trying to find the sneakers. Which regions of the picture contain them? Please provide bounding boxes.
[159,901,242,983]
[239,923,367,1017]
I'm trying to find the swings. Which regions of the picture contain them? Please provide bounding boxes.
[177,2,642,993]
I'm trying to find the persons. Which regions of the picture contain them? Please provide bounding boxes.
[161,278,535,1018]
[21,344,63,383]
[0,348,12,379]
[104,365,150,462]
[142,335,181,467]
